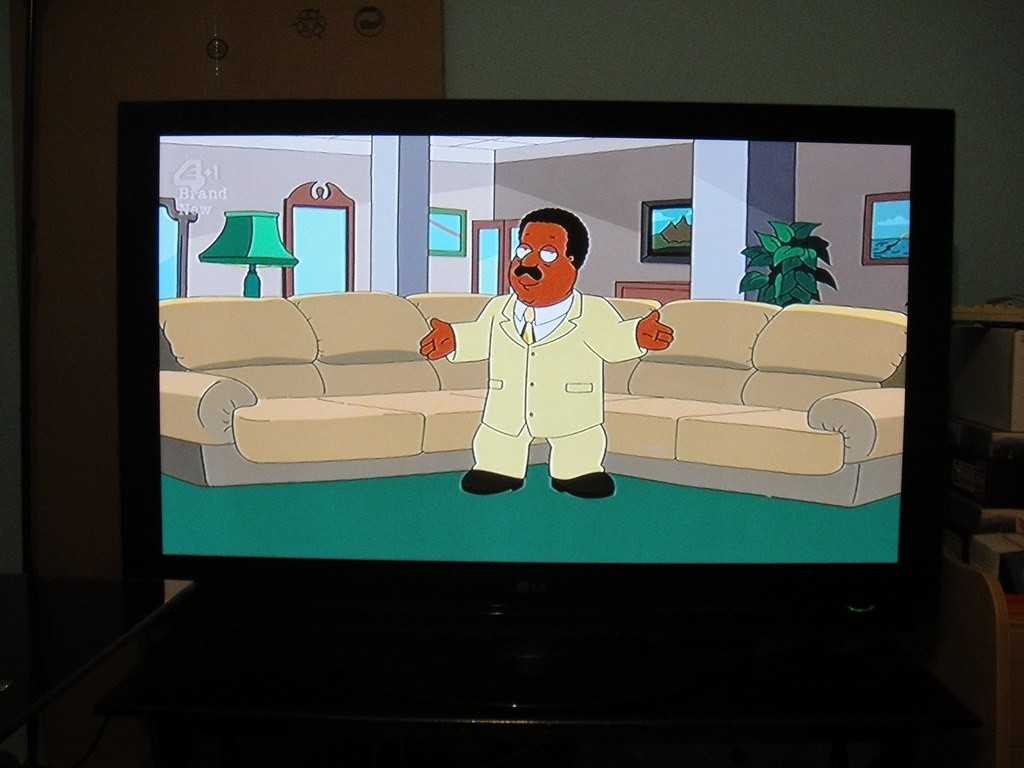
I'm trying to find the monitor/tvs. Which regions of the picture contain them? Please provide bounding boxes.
[121,98,956,595]
